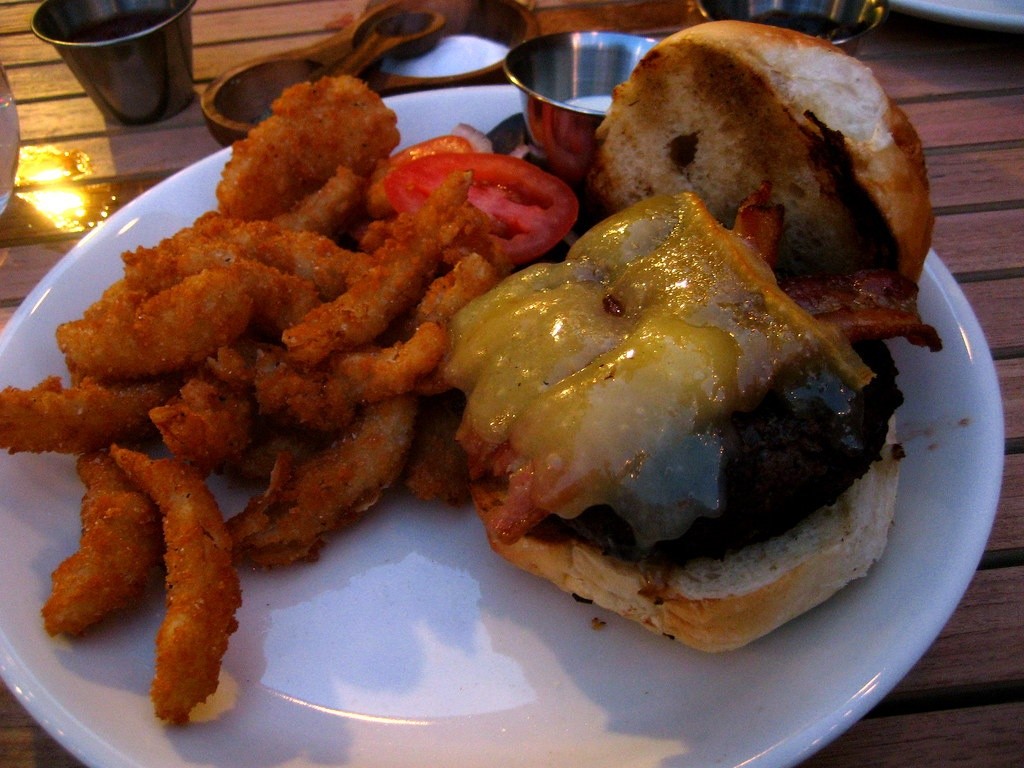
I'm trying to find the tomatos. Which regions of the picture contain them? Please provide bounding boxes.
[385,151,577,270]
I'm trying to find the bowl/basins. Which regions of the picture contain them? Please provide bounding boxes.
[29,0,200,124]
[504,30,660,183]
[697,0,884,58]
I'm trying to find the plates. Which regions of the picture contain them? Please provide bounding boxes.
[0,85,1007,768]
[888,0,1024,35]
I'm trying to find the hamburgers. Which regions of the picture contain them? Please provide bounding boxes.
[434,20,949,656]
